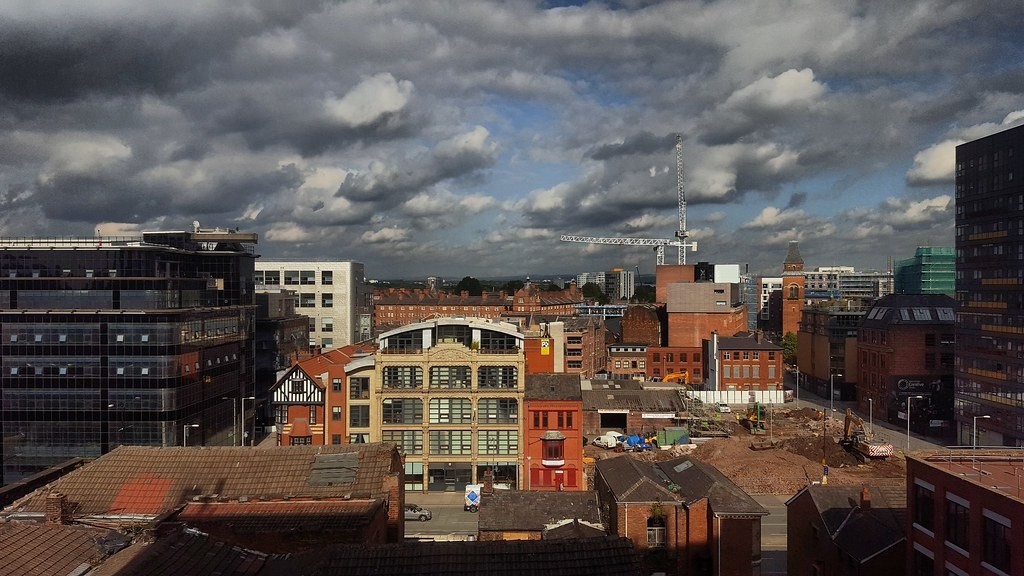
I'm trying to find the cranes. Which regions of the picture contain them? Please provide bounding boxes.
[560,134,698,265]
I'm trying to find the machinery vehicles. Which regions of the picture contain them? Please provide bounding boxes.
[746,401,767,434]
[651,370,689,384]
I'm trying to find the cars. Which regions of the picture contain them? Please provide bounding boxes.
[405,503,432,522]
[592,436,616,450]
[715,402,731,414]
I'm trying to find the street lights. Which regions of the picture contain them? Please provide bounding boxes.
[831,374,842,409]
[973,415,991,469]
[908,396,923,449]
[223,396,236,447]
[184,425,200,447]
[242,397,255,446]
[793,364,799,409]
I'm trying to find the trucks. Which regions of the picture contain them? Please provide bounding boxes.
[464,484,511,513]
